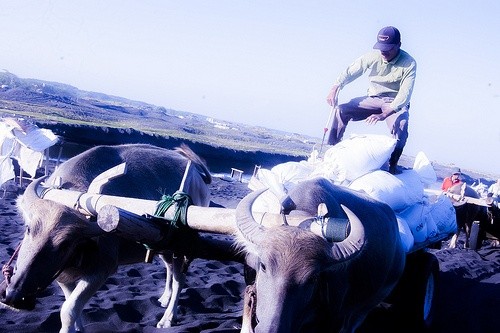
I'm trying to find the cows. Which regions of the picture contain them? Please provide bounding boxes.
[0,142,213,333]
[234,178,405,333]
[445,185,500,248]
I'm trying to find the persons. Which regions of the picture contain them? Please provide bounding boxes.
[488,177,500,198]
[325,26,417,174]
[441,167,464,194]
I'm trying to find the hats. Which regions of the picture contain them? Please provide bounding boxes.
[373,26,401,51]
[452,167,461,175]
